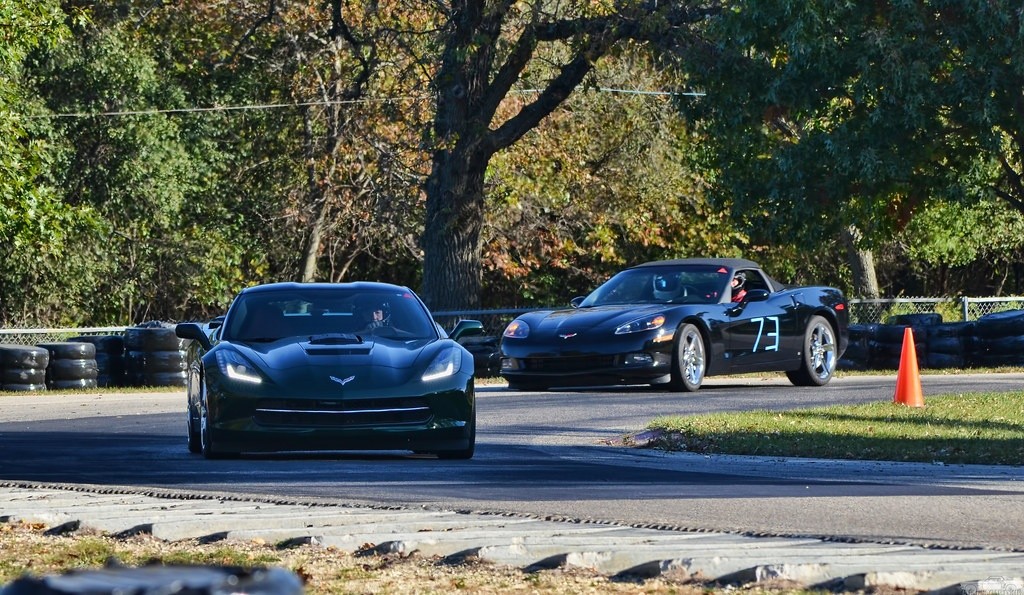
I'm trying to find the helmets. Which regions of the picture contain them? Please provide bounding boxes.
[352,294,394,329]
[730,270,749,291]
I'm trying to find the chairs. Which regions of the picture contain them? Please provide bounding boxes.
[249,303,287,342]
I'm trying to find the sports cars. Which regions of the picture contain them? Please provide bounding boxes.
[175,282,484,460]
[498,259,850,392]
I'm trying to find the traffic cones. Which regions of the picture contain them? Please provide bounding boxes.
[893,327,924,409]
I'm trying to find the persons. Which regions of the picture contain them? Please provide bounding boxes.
[348,294,414,338]
[654,272,682,300]
[705,268,746,303]
